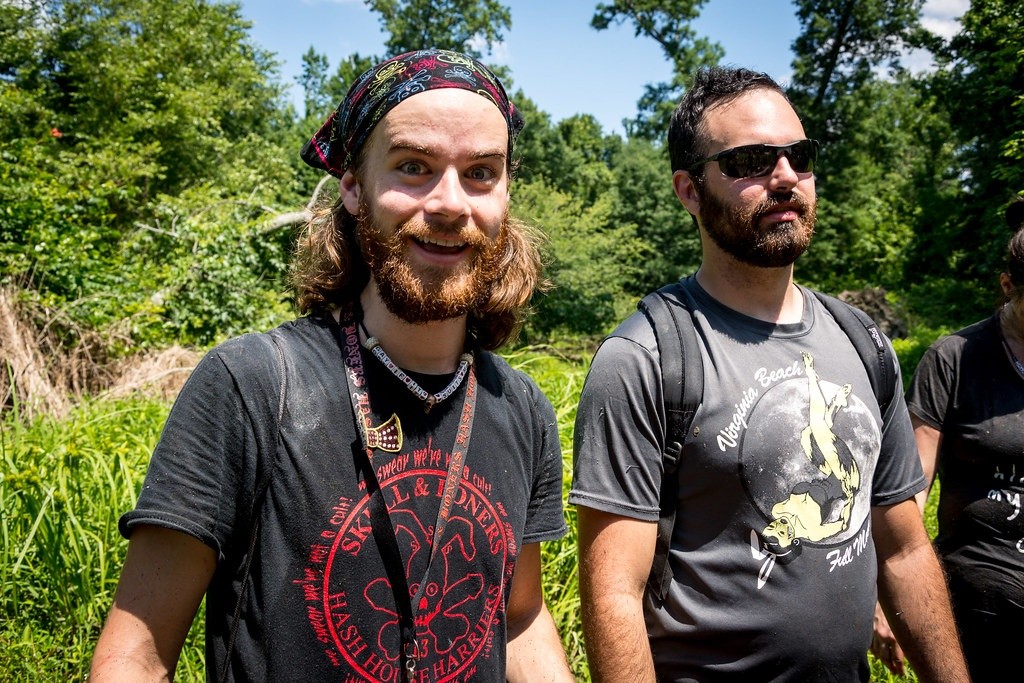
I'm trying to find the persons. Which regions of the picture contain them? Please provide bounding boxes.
[870,226,1023,683]
[568,64,971,683]
[89,48,575,683]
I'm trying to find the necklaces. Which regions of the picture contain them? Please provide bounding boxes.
[361,320,473,414]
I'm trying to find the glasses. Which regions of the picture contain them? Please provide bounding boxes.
[683,138,821,180]
